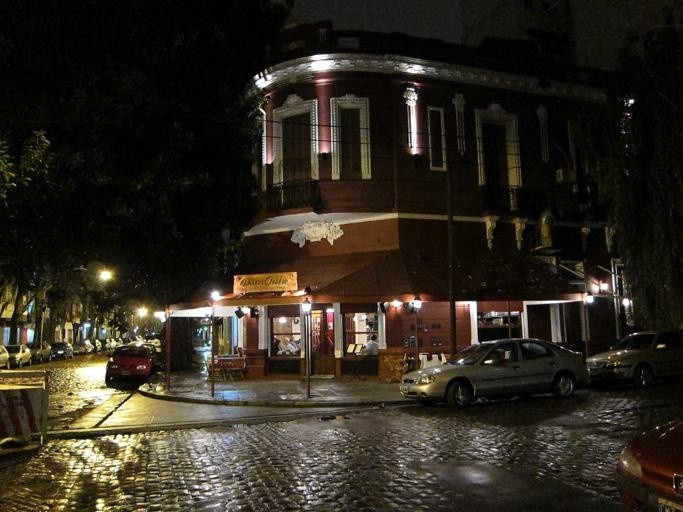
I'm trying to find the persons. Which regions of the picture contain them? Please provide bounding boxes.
[365,336,379,350]
[363,320,375,341]
[273,338,284,355]
[281,335,295,351]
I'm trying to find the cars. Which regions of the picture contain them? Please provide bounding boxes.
[615,417,682,510]
[396,337,587,408]
[584,327,683,389]
[0,332,163,387]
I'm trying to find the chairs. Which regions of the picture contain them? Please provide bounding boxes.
[206,358,245,382]
[385,354,410,384]
